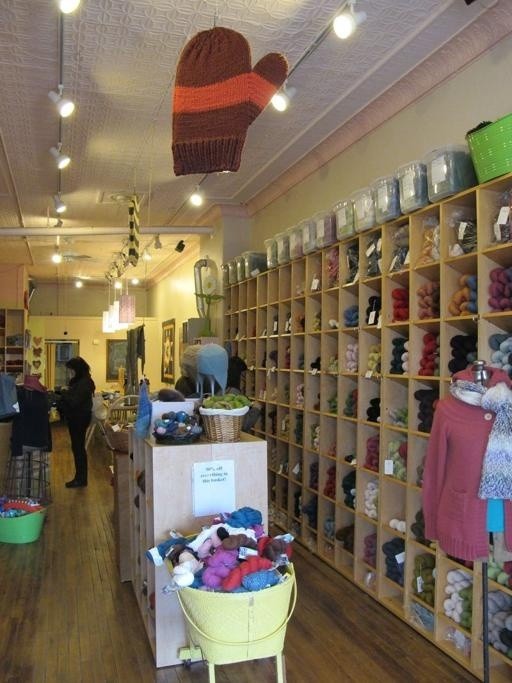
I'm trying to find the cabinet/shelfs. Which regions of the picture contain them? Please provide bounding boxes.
[0,307,26,378]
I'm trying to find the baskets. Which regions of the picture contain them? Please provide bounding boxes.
[104,395,141,452]
[198,405,250,443]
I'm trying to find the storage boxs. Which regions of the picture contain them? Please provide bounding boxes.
[394,143,475,215]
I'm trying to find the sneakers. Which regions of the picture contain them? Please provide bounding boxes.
[66,477,88,488]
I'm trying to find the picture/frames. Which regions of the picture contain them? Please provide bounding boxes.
[106,338,128,382]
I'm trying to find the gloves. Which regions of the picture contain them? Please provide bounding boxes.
[170,26,287,176]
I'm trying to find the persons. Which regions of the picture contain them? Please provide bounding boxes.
[49,356,97,488]
[218,355,262,432]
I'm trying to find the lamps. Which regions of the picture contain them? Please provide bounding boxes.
[270,0,367,112]
[46,83,76,214]
[102,270,136,333]
